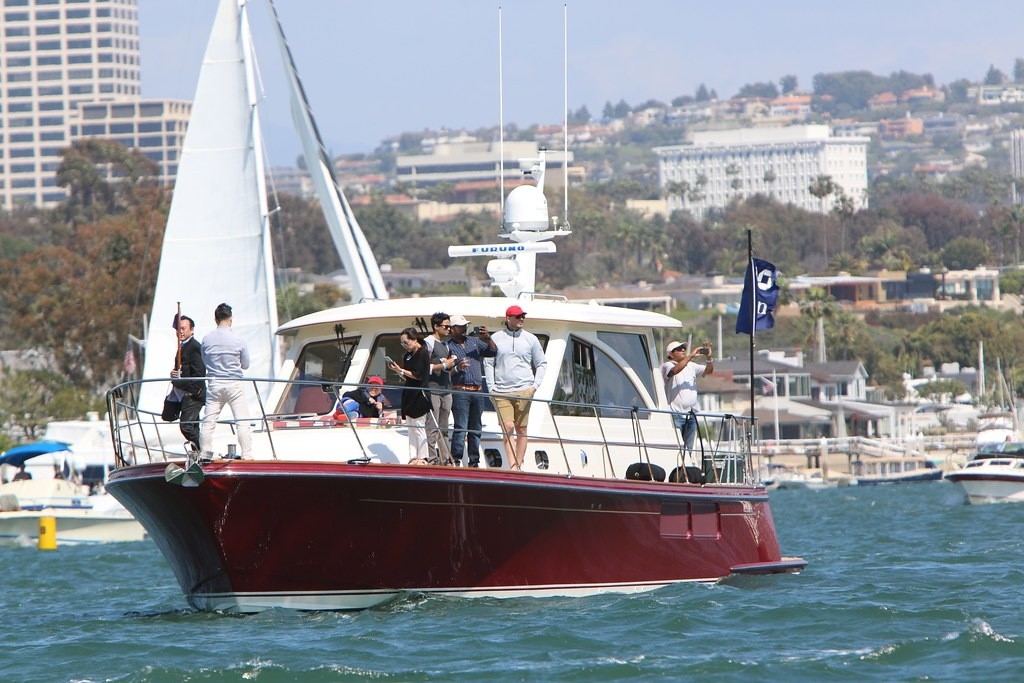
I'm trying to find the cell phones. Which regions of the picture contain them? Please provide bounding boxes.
[474,327,483,334]
[700,349,709,354]
[384,356,395,366]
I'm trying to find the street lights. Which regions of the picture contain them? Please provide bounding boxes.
[942,266,947,300]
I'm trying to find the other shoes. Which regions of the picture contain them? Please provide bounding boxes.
[410,459,428,465]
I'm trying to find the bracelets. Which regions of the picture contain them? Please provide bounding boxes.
[397,369,405,377]
[443,363,446,369]
[706,359,713,363]
[455,366,458,372]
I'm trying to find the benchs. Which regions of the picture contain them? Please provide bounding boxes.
[260,407,407,431]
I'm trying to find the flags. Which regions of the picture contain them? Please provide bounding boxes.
[763,381,771,393]
[735,256,781,334]
[120,342,137,386]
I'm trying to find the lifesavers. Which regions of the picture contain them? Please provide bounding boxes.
[273,420,337,430]
[318,413,400,428]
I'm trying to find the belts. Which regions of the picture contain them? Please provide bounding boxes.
[452,384,483,391]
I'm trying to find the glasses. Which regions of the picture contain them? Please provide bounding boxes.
[438,325,452,330]
[508,314,525,319]
[676,345,686,351]
[401,338,412,348]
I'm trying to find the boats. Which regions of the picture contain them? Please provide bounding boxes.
[694,338,1024,505]
[105,0,807,614]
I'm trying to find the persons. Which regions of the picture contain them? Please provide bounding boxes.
[662,341,714,454]
[485,306,547,471]
[551,374,637,417]
[198,302,253,459]
[424,312,455,466]
[54,464,106,495]
[321,361,341,380]
[11,464,31,482]
[333,377,384,424]
[386,328,434,465]
[446,314,499,468]
[161,315,206,450]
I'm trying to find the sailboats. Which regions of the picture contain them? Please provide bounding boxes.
[0,1,390,544]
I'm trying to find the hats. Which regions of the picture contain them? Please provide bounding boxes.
[368,377,384,386]
[506,305,528,317]
[449,314,472,327]
[666,341,688,357]
